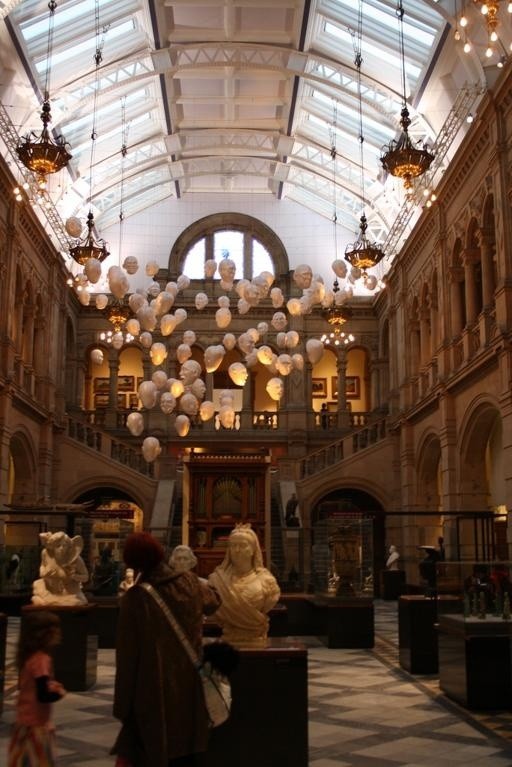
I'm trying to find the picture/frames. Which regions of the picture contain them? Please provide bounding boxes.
[138,377,144,392]
[93,378,110,392]
[331,376,360,399]
[313,378,327,398]
[129,394,138,407]
[117,376,135,391]
[328,402,351,412]
[117,394,126,408]
[95,394,109,407]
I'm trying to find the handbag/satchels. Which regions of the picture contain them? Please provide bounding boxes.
[198,662,234,729]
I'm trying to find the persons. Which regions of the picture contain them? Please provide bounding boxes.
[4,610,66,767]
[32,522,91,605]
[168,544,199,578]
[116,565,137,602]
[110,530,224,767]
[384,545,400,568]
[215,521,281,647]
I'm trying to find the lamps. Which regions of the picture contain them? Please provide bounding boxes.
[14,3,71,189]
[102,147,136,336]
[322,149,356,363]
[378,2,435,190]
[345,59,386,275]
[68,42,111,268]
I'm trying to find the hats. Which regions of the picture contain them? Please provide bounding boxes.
[123,530,166,573]
